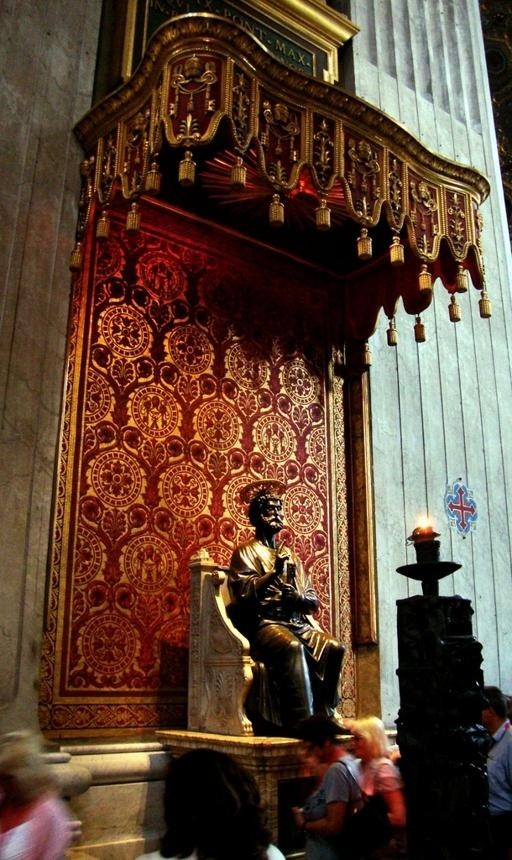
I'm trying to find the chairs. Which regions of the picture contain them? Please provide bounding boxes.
[186,547,269,735]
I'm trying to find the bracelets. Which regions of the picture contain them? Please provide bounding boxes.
[302,819,306,832]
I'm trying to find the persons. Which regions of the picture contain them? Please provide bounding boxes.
[132,746,288,860]
[228,490,352,737]
[482,685,511,858]
[289,713,369,860]
[344,716,410,860]
[1,780,84,860]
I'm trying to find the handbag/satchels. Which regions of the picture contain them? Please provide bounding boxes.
[329,794,397,859]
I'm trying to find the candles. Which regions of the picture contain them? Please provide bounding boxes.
[412,526,434,543]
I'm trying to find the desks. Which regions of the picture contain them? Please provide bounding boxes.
[153,730,356,847]
